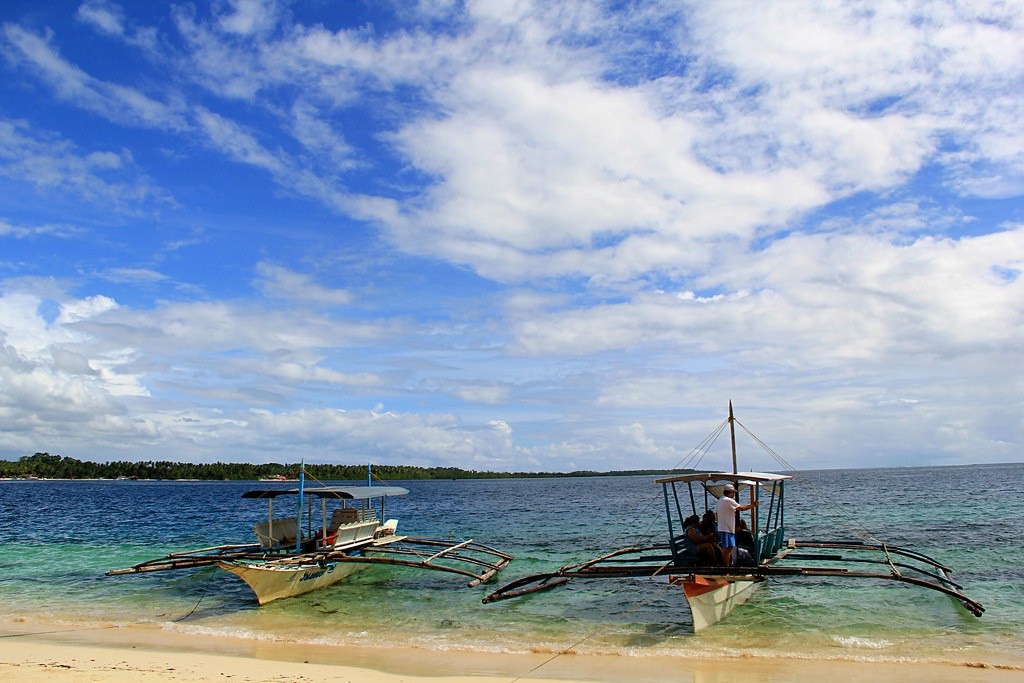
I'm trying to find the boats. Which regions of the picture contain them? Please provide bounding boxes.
[117,476,129,481]
[482,399,985,635]
[104,458,516,608]
[257,474,300,483]
[25,474,39,480]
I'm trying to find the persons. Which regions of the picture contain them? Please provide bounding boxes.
[731,519,754,566]
[685,515,718,565]
[716,484,759,567]
[702,511,717,537]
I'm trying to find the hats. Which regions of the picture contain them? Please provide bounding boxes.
[724,484,738,493]
